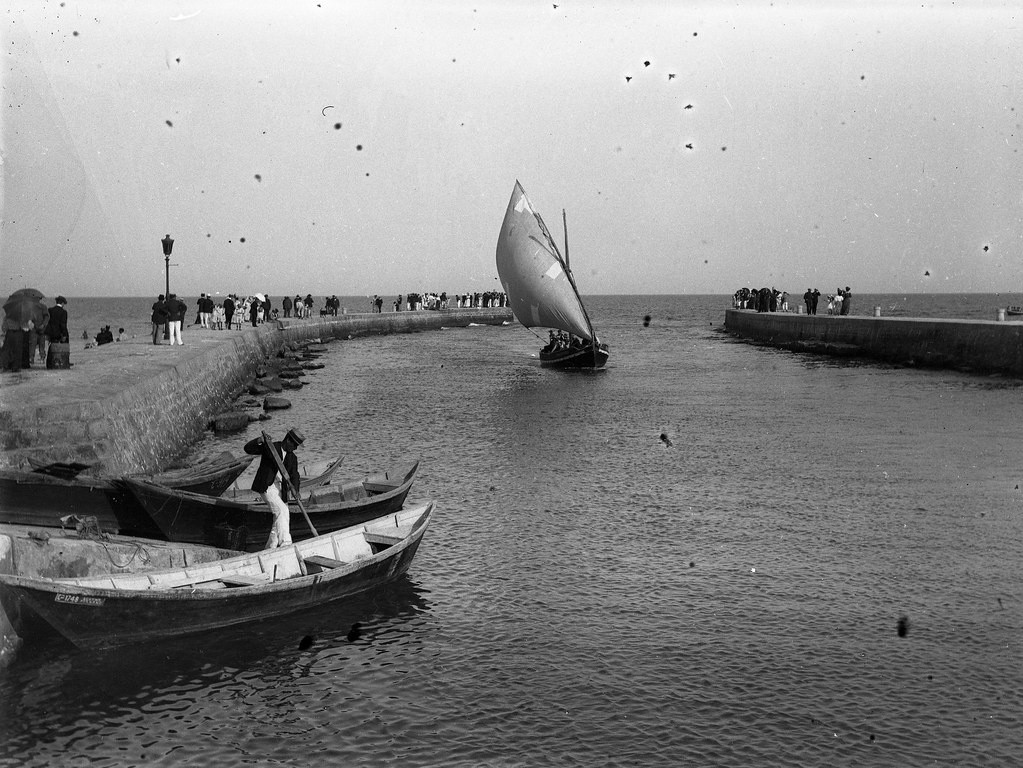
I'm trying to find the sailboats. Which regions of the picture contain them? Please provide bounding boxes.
[497,179,611,372]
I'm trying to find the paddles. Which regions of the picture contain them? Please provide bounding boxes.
[262,429,319,538]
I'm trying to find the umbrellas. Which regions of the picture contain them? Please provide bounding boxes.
[3,286,46,320]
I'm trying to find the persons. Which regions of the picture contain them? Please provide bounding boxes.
[733,287,790,313]
[393,294,402,311]
[804,288,821,315]
[152,294,187,345]
[407,291,451,311]
[283,294,314,319]
[320,294,340,317]
[371,295,383,313]
[244,428,307,549]
[0,315,50,372]
[548,329,569,352]
[456,291,510,309]
[828,287,852,315]
[197,293,279,331]
[95,325,128,345]
[46,296,74,366]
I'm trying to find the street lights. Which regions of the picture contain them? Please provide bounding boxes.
[160,234,174,340]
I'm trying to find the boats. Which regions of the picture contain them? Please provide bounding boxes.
[119,460,422,553]
[0,451,348,536]
[1,499,438,655]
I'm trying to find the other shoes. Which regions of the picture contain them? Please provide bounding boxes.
[12,368,21,372]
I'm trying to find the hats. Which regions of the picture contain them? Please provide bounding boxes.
[285,428,307,448]
[208,294,211,297]
[55,296,67,304]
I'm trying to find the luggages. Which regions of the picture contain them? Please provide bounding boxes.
[46,340,69,369]
